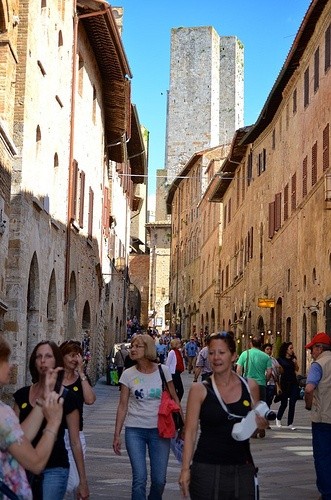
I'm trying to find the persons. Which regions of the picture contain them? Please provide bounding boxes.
[0,314,331,500]
[112,334,185,500]
[178,331,270,500]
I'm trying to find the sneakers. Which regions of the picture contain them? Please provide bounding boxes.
[288,425,297,431]
[275,418,282,428]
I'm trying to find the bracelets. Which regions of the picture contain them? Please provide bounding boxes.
[181,468,189,472]
[80,376,87,382]
[43,428,57,436]
[35,400,43,408]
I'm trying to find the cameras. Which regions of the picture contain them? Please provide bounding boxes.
[264,410,276,420]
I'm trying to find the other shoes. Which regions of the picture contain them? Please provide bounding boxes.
[193,371,195,374]
[250,434,258,439]
[259,432,266,438]
[186,368,187,370]
[189,371,191,374]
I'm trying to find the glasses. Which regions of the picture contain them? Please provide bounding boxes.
[130,344,144,348]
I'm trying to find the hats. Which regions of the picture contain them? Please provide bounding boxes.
[306,332,331,348]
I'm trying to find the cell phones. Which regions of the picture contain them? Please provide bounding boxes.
[55,370,64,394]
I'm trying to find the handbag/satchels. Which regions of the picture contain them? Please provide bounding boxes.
[157,363,184,439]
